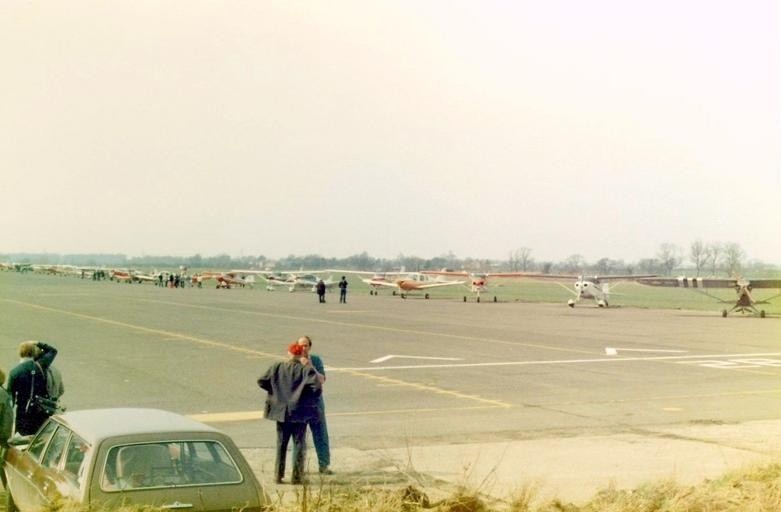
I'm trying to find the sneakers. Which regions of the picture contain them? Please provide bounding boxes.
[319,467,331,474]
[275,478,302,484]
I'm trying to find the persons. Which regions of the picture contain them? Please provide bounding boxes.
[317,280,326,303]
[92,270,105,281]
[339,276,348,303]
[0,341,65,446]
[197,273,203,288]
[257,336,334,485]
[158,271,185,289]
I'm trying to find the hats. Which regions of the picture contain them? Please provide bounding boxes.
[287,343,304,356]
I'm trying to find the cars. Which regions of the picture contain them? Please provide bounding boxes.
[0,407,276,512]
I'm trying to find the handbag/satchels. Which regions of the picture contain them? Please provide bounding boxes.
[25,395,57,417]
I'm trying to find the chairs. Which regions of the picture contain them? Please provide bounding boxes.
[118,445,178,491]
